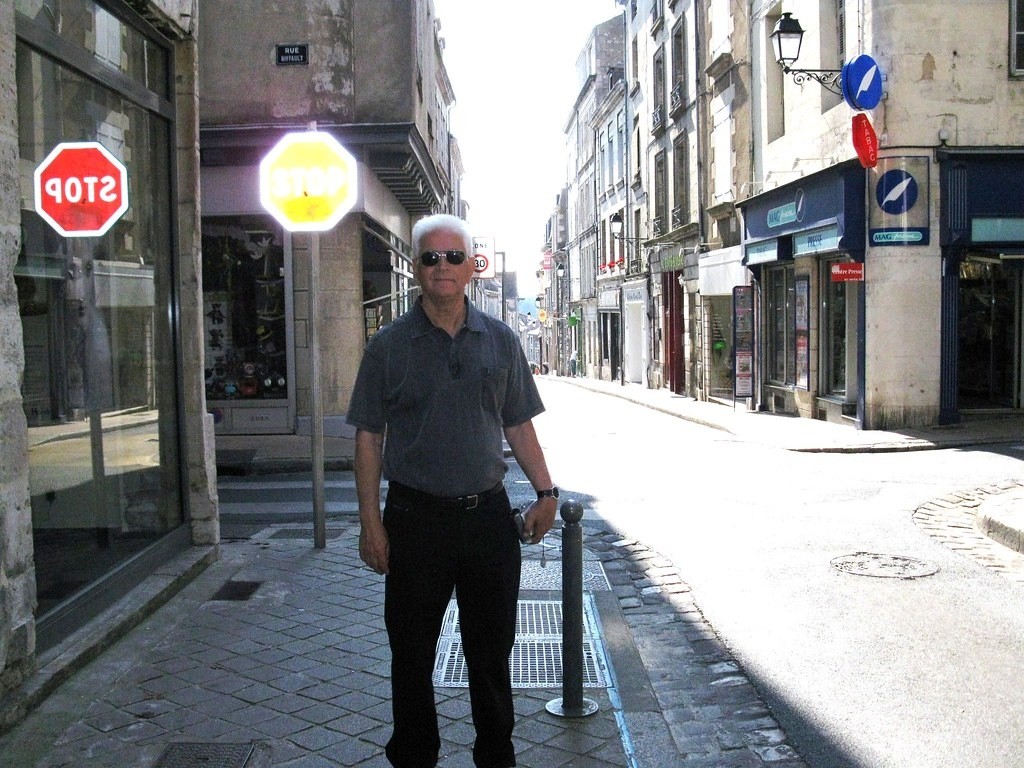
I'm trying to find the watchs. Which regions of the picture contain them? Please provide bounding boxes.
[537,486,560,500]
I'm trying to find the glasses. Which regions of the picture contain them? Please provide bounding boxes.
[412,250,468,266]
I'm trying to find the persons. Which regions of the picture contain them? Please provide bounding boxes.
[542,362,549,375]
[570,351,578,378]
[345,214,561,767]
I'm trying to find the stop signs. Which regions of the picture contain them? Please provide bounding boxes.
[256,131,357,235]
[34,141,130,240]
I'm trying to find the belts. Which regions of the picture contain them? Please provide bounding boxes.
[387,482,503,510]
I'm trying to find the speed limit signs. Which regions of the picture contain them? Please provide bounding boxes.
[471,238,496,279]
